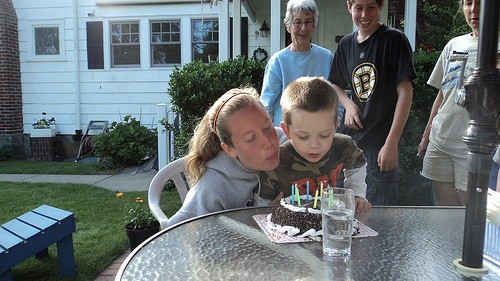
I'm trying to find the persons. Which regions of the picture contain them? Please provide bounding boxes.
[258,0,345,145]
[253,76,373,215]
[327,0,416,206]
[158,86,288,231]
[416,0,500,206]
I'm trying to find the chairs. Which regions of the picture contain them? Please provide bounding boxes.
[148,156,190,230]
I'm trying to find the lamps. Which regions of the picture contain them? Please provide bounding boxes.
[259,20,270,37]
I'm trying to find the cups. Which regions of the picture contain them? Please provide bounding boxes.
[321,187,356,258]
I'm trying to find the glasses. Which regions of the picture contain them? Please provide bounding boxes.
[291,19,314,27]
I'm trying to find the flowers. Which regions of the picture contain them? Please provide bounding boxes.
[31,112,55,129]
[116,193,156,229]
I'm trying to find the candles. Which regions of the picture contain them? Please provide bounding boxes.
[292,180,333,209]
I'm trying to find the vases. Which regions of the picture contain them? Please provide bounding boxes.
[30,129,55,137]
[125,222,160,253]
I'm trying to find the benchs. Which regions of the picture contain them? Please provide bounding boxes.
[0,205,76,281]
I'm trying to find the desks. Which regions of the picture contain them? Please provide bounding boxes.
[114,205,500,281]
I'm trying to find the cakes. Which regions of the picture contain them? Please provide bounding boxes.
[266,193,348,239]
